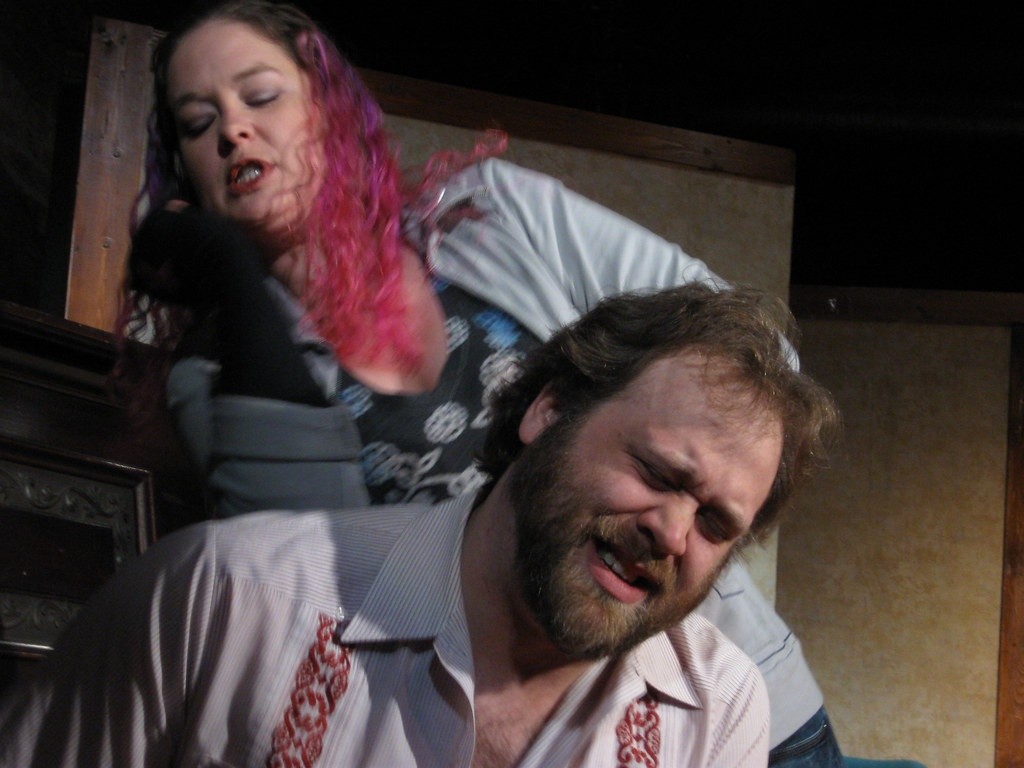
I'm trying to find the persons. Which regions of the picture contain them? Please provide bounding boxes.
[110,9,844,768]
[0,282,843,768]
